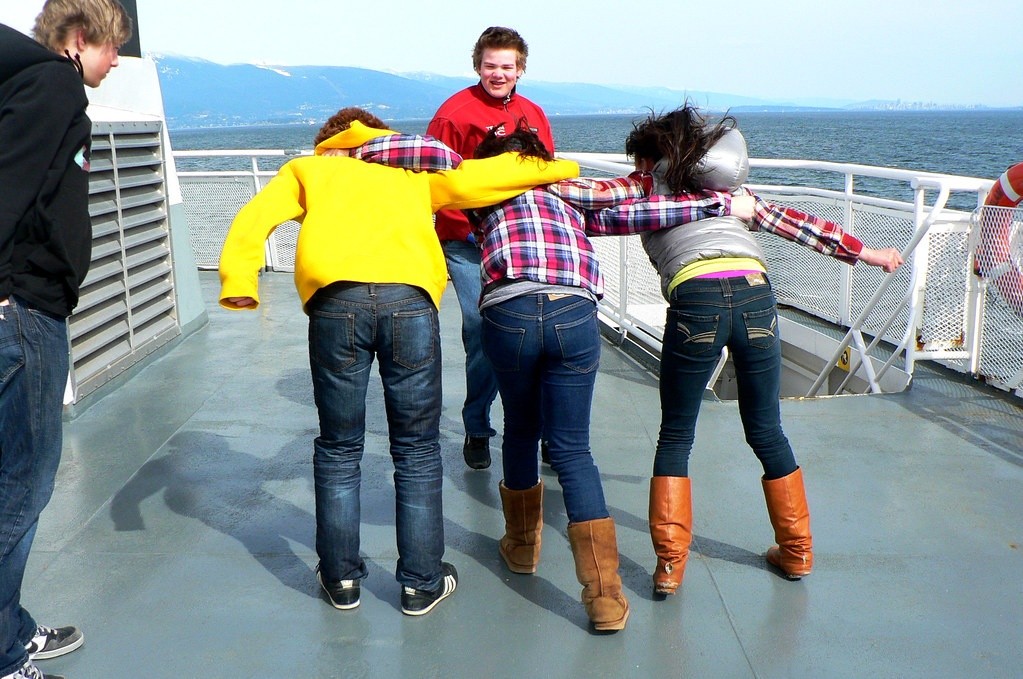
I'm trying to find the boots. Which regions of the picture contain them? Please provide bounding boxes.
[568,517,631,630]
[648,476,693,596]
[497,477,542,574]
[762,465,814,579]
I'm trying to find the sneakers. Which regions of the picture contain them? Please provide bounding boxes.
[316,560,361,610]
[1,663,68,679]
[462,434,491,470]
[20,626,87,662]
[541,441,550,463]
[399,561,459,616]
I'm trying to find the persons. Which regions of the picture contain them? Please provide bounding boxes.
[320,115,756,631]
[218,109,580,616]
[546,99,904,594]
[426,27,555,469]
[0,0,132,679]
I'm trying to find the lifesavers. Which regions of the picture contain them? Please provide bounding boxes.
[980,161,1023,317]
